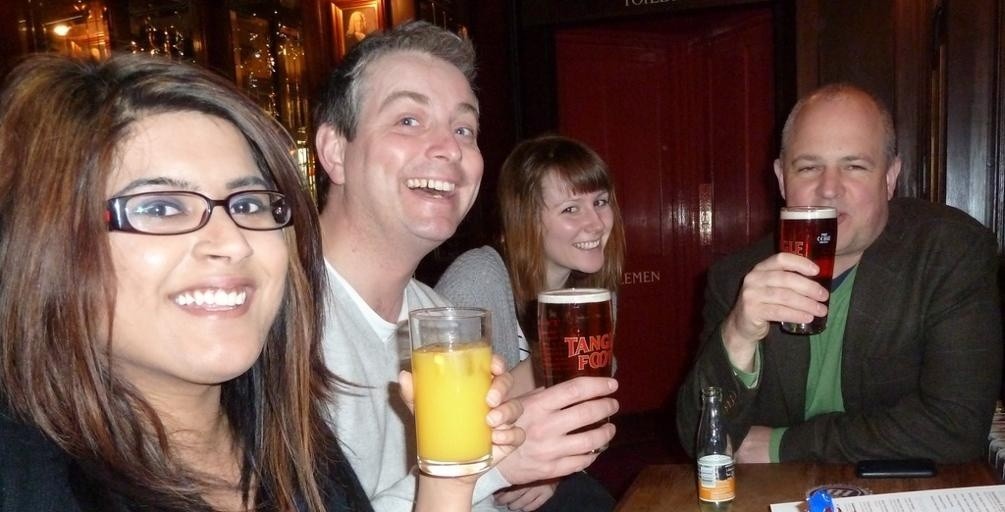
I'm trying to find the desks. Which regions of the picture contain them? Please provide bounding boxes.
[609,418,1005,511]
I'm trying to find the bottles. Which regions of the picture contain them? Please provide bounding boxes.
[126,23,186,60]
[696,385,736,512]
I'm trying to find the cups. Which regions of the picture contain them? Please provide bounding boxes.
[779,205,838,337]
[535,287,615,452]
[407,306,493,480]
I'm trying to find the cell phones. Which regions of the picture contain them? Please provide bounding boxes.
[859,458,934,479]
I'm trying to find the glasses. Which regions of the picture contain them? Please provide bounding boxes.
[96,191,298,237]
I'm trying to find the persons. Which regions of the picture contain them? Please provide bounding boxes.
[433,134,628,512]
[0,54,525,512]
[313,19,622,512]
[676,82,1005,465]
